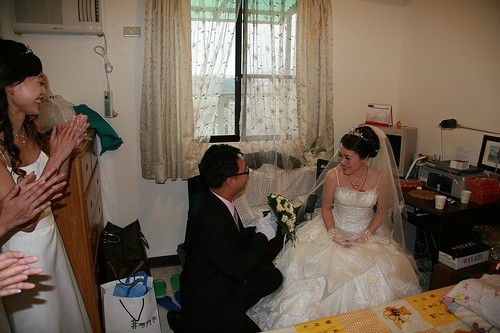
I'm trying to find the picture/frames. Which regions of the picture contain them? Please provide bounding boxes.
[477,134,500,174]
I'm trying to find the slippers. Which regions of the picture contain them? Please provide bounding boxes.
[156,291,182,312]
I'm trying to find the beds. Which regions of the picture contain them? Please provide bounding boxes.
[260,274,500,333]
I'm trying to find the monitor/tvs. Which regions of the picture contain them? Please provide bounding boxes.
[375,125,418,177]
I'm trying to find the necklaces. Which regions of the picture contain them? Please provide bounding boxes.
[348,167,368,190]
[16,129,26,143]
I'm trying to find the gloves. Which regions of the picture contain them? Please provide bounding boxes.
[254,212,279,241]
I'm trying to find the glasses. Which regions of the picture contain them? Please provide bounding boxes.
[226,167,250,179]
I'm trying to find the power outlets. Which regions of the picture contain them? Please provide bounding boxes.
[123,27,141,37]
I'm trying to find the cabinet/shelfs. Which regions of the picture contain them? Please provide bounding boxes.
[51,138,104,333]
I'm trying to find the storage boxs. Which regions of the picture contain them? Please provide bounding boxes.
[438,237,490,271]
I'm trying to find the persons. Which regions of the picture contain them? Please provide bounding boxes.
[245,124,423,332]
[0,37,93,333]
[167,144,284,333]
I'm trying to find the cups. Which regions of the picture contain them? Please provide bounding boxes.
[460,189,472,204]
[435,195,447,210]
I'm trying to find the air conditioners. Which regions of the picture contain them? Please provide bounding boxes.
[8,0,118,119]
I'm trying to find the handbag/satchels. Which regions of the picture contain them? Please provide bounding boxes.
[100,273,165,333]
[95,219,152,280]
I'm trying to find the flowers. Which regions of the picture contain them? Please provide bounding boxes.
[267,194,300,251]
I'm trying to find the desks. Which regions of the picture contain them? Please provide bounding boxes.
[375,177,476,260]
